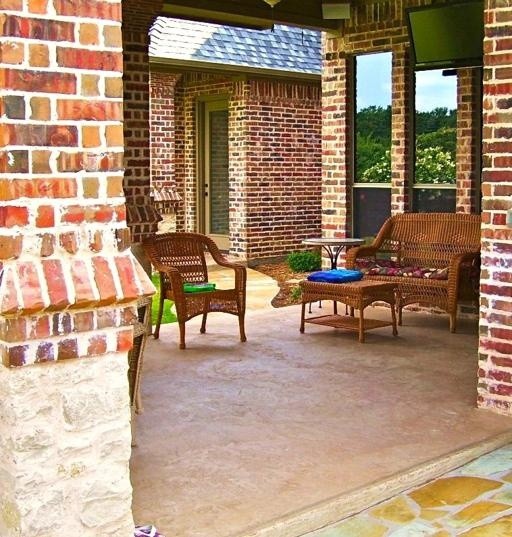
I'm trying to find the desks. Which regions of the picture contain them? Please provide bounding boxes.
[302,237,365,270]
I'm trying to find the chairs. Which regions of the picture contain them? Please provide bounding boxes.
[142,232,247,350]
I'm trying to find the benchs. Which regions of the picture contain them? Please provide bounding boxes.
[345,213,481,333]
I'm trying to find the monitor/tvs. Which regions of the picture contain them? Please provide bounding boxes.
[402,0,484,73]
